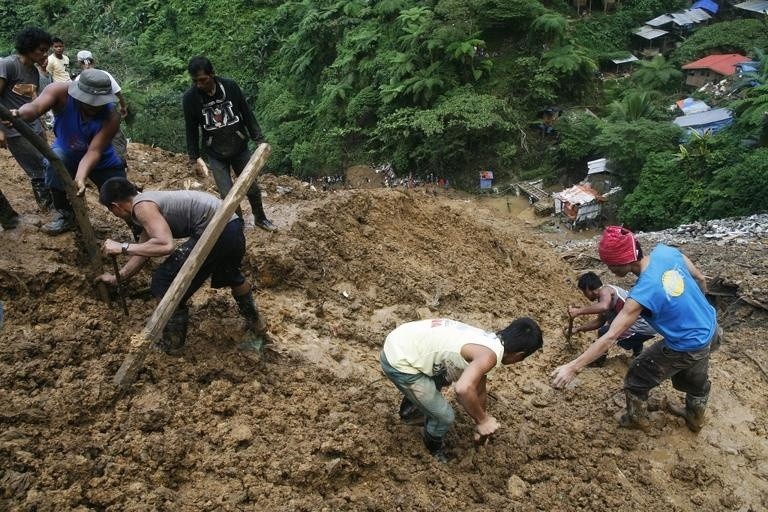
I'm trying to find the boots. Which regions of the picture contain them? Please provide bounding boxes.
[617,388,652,434]
[232,283,268,333]
[423,428,452,468]
[398,393,425,422]
[152,303,189,356]
[631,341,644,358]
[28,177,55,214]
[245,190,281,233]
[587,351,607,368]
[667,392,709,434]
[221,194,243,220]
[40,189,77,236]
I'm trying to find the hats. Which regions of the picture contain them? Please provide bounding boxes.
[78,48,95,68]
[67,68,121,107]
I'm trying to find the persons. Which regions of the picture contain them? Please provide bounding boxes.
[0,27,52,215]
[35,47,52,97]
[19,69,143,242]
[78,49,128,166]
[552,224,718,431]
[306,161,450,188]
[185,54,277,234]
[380,318,543,455]
[94,176,271,354]
[567,272,655,365]
[48,38,72,88]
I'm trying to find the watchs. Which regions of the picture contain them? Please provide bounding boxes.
[121,241,129,254]
